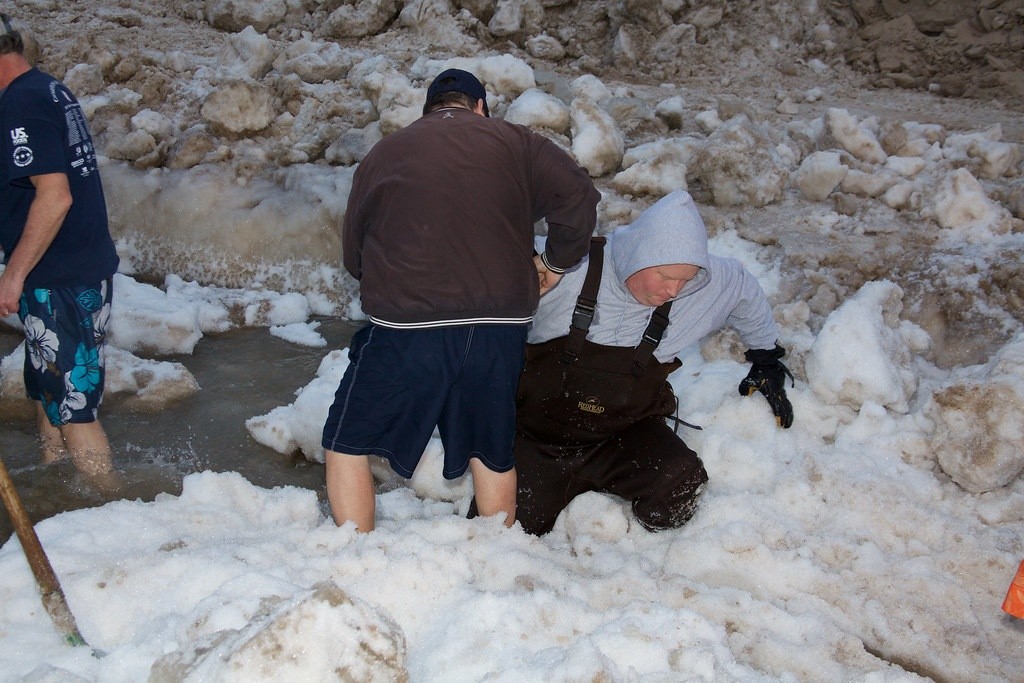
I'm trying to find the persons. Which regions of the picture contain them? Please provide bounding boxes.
[1,9,117,489]
[463,190,798,534]
[319,67,604,534]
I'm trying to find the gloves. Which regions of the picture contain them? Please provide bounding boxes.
[738,344,796,428]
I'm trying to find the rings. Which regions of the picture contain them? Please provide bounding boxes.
[2,309,10,316]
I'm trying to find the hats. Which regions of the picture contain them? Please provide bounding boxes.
[427,68,490,118]
[0,13,21,43]
